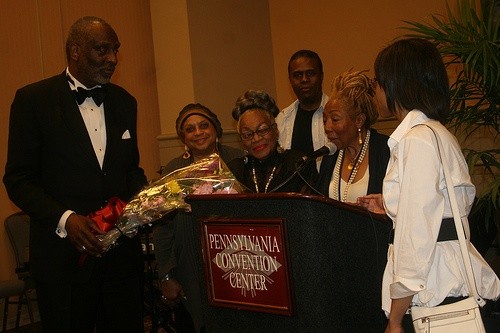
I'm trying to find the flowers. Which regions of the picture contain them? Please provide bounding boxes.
[80,153,243,258]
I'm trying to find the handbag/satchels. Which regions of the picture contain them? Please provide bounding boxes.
[411,296,486,333]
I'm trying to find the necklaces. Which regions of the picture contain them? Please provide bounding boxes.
[251,158,277,193]
[337,128,370,202]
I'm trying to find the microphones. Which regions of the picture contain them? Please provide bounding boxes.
[297,142,337,164]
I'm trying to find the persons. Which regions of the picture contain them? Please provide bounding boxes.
[357,38,496,333]
[154,48,391,307]
[2,15,148,332]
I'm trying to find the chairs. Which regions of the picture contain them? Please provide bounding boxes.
[0,211,41,333]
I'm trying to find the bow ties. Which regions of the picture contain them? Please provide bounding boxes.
[72,87,106,108]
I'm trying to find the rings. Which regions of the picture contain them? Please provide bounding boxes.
[79,244,86,252]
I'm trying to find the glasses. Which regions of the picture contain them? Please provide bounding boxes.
[240,123,275,141]
